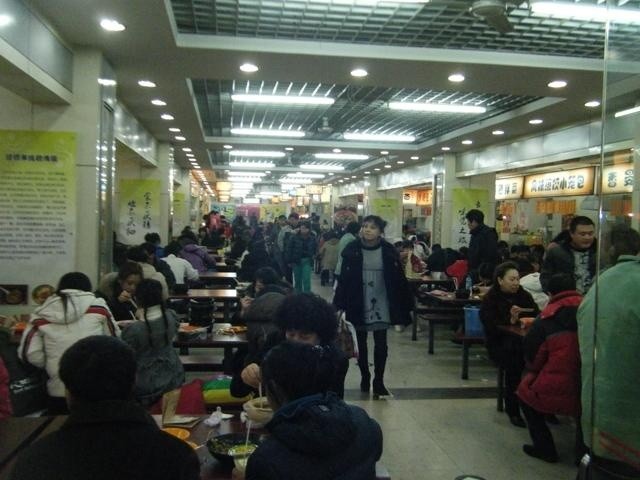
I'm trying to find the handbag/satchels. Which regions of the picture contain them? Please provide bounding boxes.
[10,370,47,415]
[334,309,359,360]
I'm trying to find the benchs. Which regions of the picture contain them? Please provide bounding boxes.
[412,305,465,340]
[454,336,486,381]
[179,354,232,373]
[422,314,463,354]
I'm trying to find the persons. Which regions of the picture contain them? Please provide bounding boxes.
[459,266,493,297]
[246,285,289,349]
[124,278,182,406]
[283,222,316,293]
[514,257,550,310]
[480,262,561,428]
[576,251,640,479]
[518,273,589,466]
[239,238,283,281]
[231,290,349,405]
[176,235,214,273]
[230,340,383,479]
[335,216,413,396]
[233,267,291,323]
[19,274,124,414]
[182,210,288,251]
[278,215,300,284]
[306,212,350,237]
[608,223,638,263]
[9,334,201,480]
[160,245,198,287]
[93,266,143,319]
[336,223,362,283]
[318,237,342,285]
[496,231,570,265]
[539,217,607,298]
[464,209,500,287]
[396,232,472,289]
[112,229,174,298]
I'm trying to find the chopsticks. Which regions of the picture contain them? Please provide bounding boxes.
[123,290,139,310]
[259,381,263,408]
[512,308,534,312]
[477,281,484,286]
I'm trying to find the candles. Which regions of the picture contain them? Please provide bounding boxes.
[425,294,488,308]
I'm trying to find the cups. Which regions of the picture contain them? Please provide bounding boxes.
[227,444,257,477]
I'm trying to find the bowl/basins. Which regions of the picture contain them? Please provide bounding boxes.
[160,428,191,441]
[519,317,535,326]
[243,396,276,425]
[206,432,270,465]
[117,319,136,331]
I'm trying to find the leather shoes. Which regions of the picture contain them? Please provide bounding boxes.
[373,380,393,399]
[360,378,371,393]
[523,444,558,463]
[509,412,527,429]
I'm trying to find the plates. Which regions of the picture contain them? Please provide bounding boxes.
[222,414,234,419]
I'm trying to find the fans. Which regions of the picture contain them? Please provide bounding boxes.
[424,0,526,35]
[318,117,332,135]
